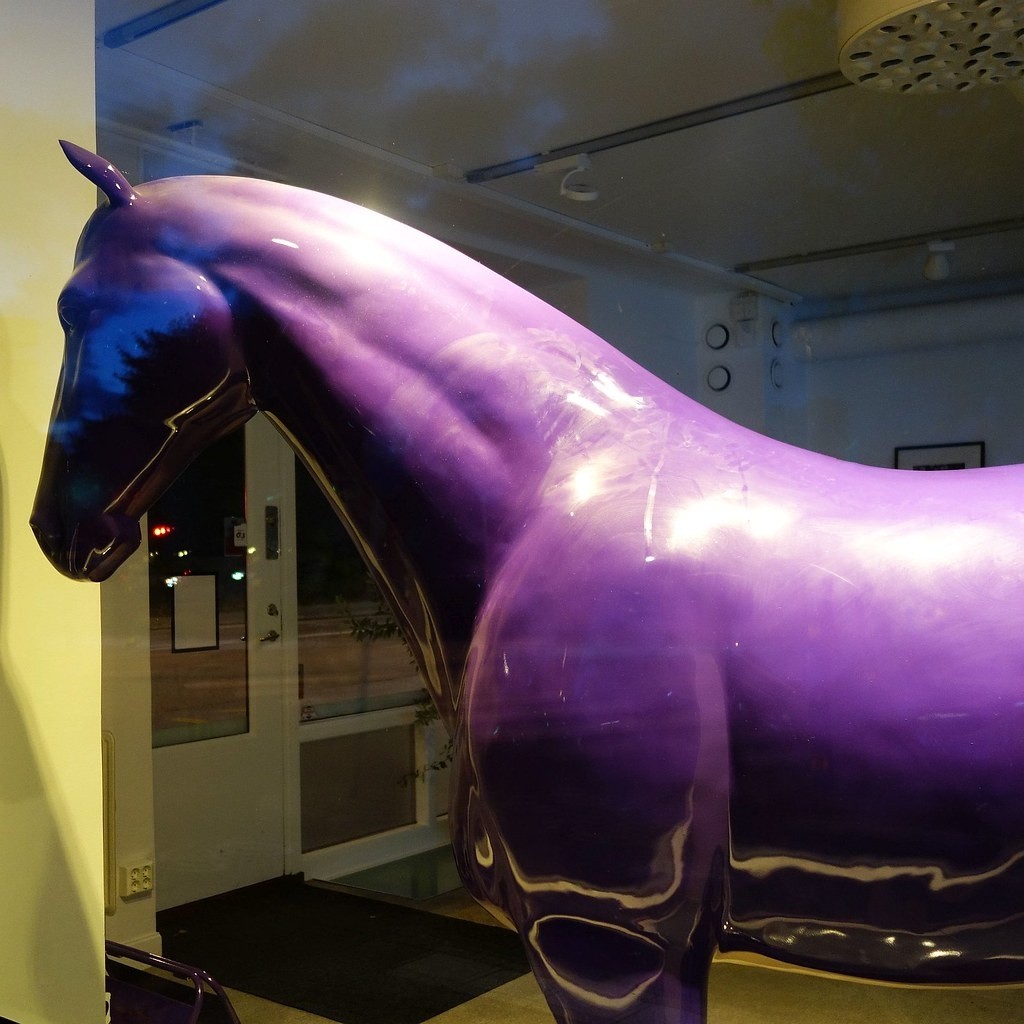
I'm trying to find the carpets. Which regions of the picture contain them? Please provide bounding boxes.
[156,872,534,1024]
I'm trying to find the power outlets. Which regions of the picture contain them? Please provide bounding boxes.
[119,859,154,896]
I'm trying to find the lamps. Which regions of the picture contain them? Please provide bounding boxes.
[835,0,1024,95]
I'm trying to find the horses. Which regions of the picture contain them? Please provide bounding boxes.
[30,138,1024,1023]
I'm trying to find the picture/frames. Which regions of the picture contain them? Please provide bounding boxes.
[894,441,986,473]
